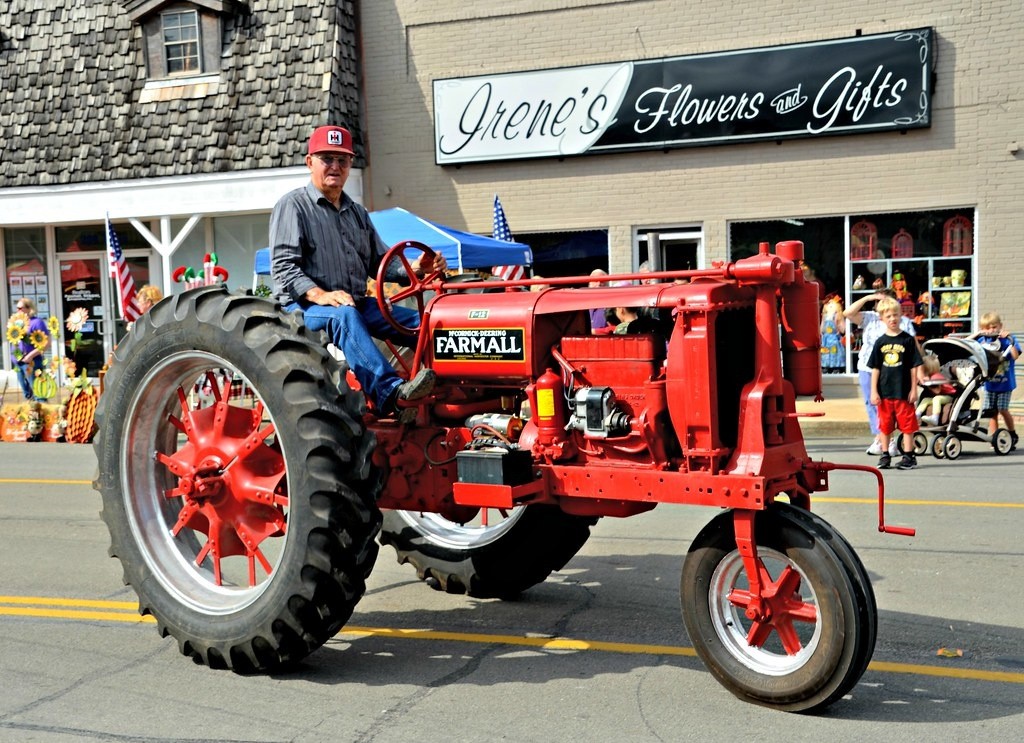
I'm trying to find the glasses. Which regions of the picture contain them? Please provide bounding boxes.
[312,154,351,169]
[877,300,879,303]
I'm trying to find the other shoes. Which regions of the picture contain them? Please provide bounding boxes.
[921,414,939,425]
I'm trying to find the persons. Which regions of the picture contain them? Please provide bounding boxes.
[963,311,1022,450]
[820,293,846,374]
[136,284,162,314]
[269,127,448,422]
[10,298,50,402]
[531,260,691,335]
[843,289,960,470]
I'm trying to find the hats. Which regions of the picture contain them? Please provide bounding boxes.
[308,126,355,156]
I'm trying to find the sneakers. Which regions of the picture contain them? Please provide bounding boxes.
[895,455,918,469]
[392,368,436,424]
[888,441,901,456]
[866,438,882,455]
[877,454,891,469]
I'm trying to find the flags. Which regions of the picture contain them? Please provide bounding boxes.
[492,194,530,290]
[106,209,144,323]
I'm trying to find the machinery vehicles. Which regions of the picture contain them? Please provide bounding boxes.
[88,235,920,716]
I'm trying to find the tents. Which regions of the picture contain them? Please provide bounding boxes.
[252,208,532,296]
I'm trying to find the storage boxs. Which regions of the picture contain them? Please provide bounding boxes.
[0,404,32,441]
[38,405,67,443]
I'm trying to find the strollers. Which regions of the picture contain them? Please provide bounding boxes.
[896,330,1014,461]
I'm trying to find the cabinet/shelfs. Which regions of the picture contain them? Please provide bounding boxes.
[852,255,975,322]
